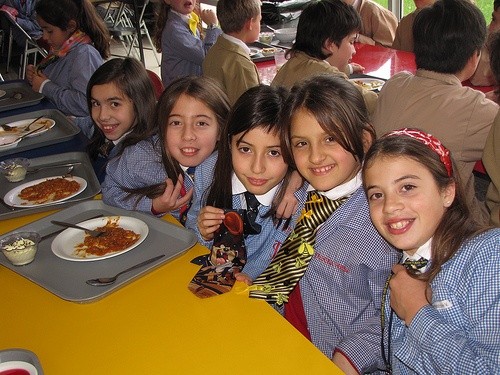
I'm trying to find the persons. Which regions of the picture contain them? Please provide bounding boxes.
[100,77,302,250]
[154,0,220,89]
[203,0,263,105]
[393,0,443,53]
[186,84,313,282]
[234,76,404,360]
[343,0,399,47]
[70,54,155,183]
[333,128,500,375]
[24,0,109,140]
[270,0,378,120]
[370,0,500,228]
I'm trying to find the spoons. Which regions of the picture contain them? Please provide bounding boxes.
[86,255,165,286]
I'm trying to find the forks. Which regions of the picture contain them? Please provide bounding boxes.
[50,219,107,238]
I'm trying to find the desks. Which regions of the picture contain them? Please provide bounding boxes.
[257,41,417,87]
[0,79,345,375]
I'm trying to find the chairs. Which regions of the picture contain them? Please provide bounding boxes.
[0,0,162,81]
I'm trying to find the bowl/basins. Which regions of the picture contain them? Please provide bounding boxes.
[274,28,297,43]
[0,135,22,151]
[262,48,279,55]
[0,157,31,183]
[0,231,40,266]
[259,31,274,43]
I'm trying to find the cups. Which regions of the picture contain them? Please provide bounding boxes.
[274,51,289,72]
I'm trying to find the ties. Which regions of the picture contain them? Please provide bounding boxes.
[249,190,349,302]
[180,167,195,226]
[187,192,260,299]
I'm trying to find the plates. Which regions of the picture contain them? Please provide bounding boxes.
[350,77,386,90]
[0,361,37,375]
[250,48,259,56]
[0,118,56,138]
[4,175,88,208]
[52,216,149,262]
[0,89,6,97]
